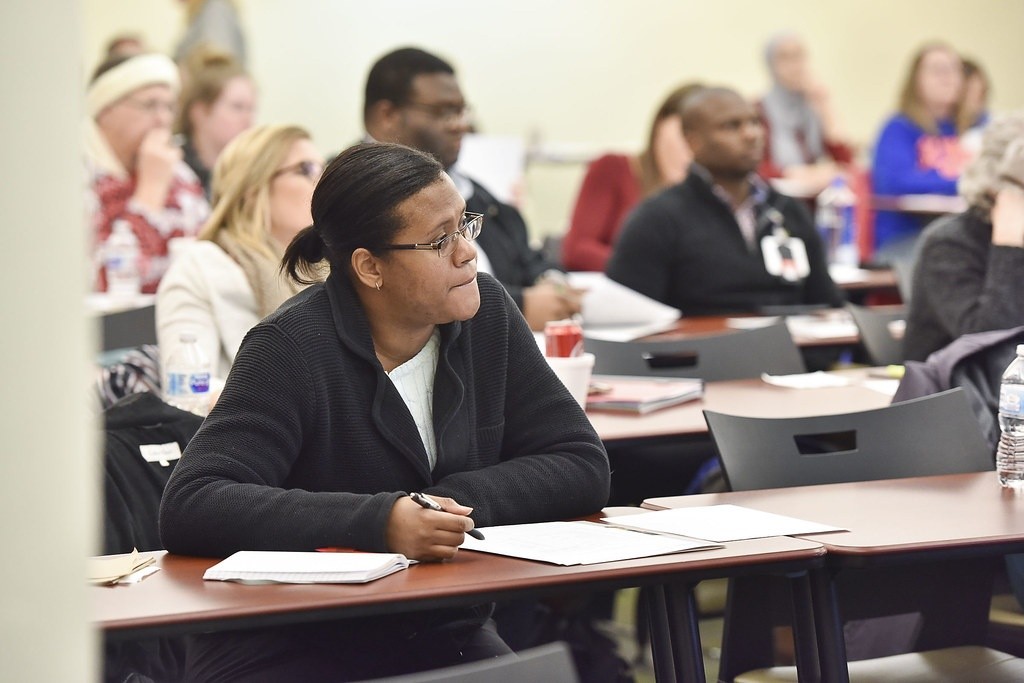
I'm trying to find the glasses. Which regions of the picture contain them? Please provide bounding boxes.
[370,211,485,257]
[272,159,322,182]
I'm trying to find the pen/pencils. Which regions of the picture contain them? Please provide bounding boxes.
[410,492,485,541]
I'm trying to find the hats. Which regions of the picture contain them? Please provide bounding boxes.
[85,52,177,121]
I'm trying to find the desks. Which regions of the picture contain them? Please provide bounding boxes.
[865,191,970,219]
[583,368,900,455]
[831,262,900,290]
[640,303,909,355]
[640,470,1024,683]
[98,514,829,683]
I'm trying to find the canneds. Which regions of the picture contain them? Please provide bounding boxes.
[544,318,584,357]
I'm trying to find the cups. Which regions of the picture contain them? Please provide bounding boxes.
[546,352,596,414]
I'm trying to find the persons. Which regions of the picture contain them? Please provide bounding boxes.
[158,140,612,683]
[754,35,856,179]
[364,48,588,329]
[902,118,1024,648]
[154,128,332,420]
[85,38,254,296]
[866,41,994,270]
[605,88,844,317]
[563,82,709,272]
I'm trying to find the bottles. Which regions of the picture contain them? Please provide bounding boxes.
[160,330,211,418]
[102,218,142,307]
[994,343,1024,490]
[813,178,862,275]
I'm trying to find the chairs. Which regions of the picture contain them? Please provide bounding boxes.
[101,198,1024,683]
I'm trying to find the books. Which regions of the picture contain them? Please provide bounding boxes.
[588,373,705,415]
[202,550,421,585]
